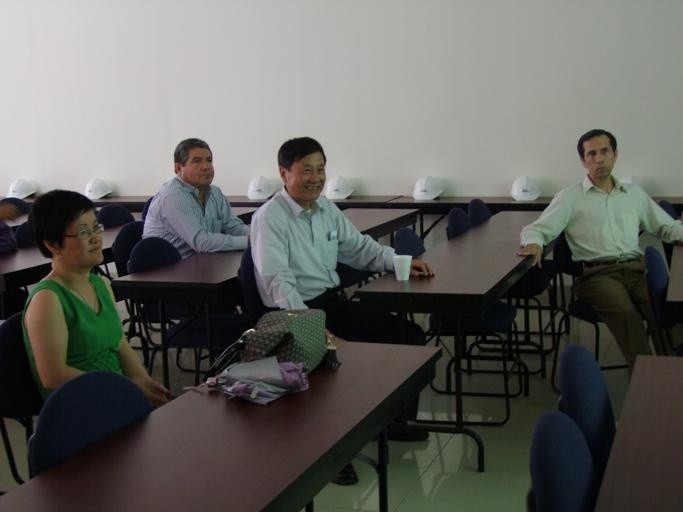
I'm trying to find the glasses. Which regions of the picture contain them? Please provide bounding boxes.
[63,224,104,239]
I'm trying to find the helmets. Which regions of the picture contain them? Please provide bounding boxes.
[7,180,36,199]
[248,176,275,200]
[326,176,353,199]
[511,176,540,200]
[414,177,444,200]
[85,178,112,200]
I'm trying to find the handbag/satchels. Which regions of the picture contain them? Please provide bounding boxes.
[239,308,327,377]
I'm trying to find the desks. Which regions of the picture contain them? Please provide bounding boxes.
[594,354,683,512]
[0,198,564,512]
[666,246,683,306]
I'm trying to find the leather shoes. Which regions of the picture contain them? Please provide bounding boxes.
[331,464,358,485]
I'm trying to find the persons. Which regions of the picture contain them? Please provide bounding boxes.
[514,129,682,381]
[247,137,434,483]
[21,191,176,410]
[143,137,254,314]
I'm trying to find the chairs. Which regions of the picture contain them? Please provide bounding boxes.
[556,344,615,471]
[645,244,683,354]
[657,196,678,219]
[528,410,599,511]
[549,240,626,402]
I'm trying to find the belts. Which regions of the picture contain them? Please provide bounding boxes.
[585,258,634,267]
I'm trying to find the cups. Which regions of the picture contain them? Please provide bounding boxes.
[393,254,413,281]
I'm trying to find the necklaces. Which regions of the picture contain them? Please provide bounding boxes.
[54,270,98,313]
